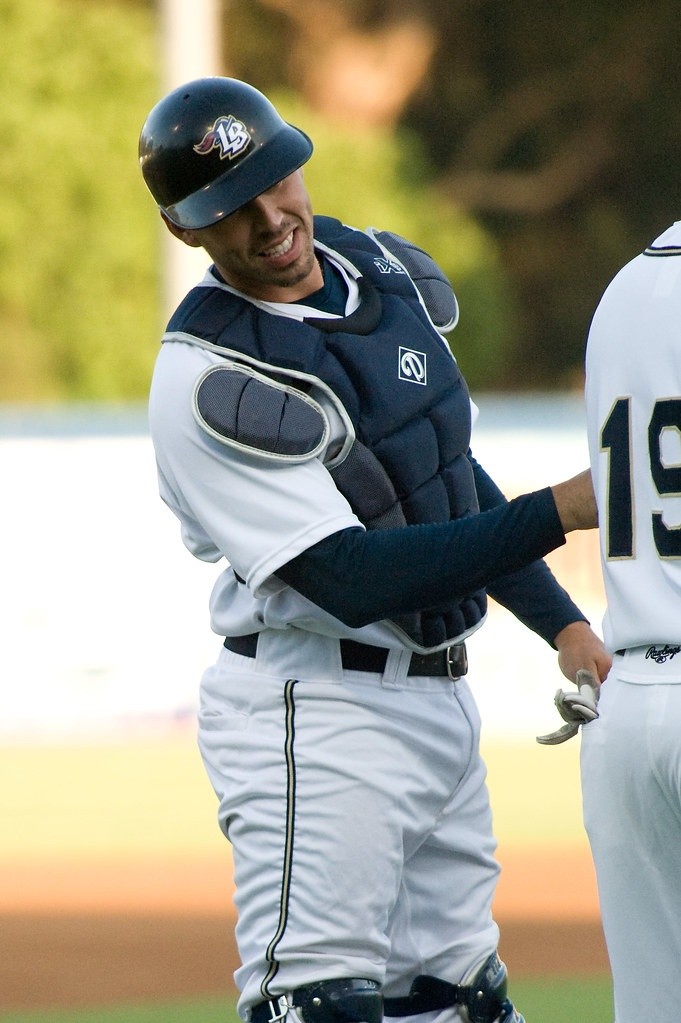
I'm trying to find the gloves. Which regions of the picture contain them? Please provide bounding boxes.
[535,669,600,745]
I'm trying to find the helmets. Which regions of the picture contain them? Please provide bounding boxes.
[138,76,315,232]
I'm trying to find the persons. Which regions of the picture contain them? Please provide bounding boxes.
[579,218,681,1022]
[139,77,612,1023]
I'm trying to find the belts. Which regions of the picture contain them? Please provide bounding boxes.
[223,631,469,682]
